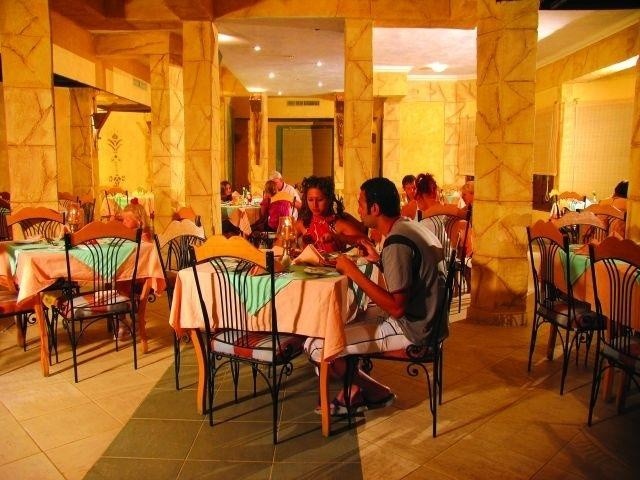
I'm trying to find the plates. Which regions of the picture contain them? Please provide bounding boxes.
[303,266,336,276]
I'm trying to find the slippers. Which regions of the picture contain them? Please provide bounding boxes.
[362,393,398,412]
[315,398,368,417]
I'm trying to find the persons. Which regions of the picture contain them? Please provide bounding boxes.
[594,181,629,237]
[220,181,233,202]
[397,173,474,241]
[251,171,303,249]
[275,176,380,263]
[304,179,446,415]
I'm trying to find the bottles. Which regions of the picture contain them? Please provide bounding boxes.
[231,186,253,206]
